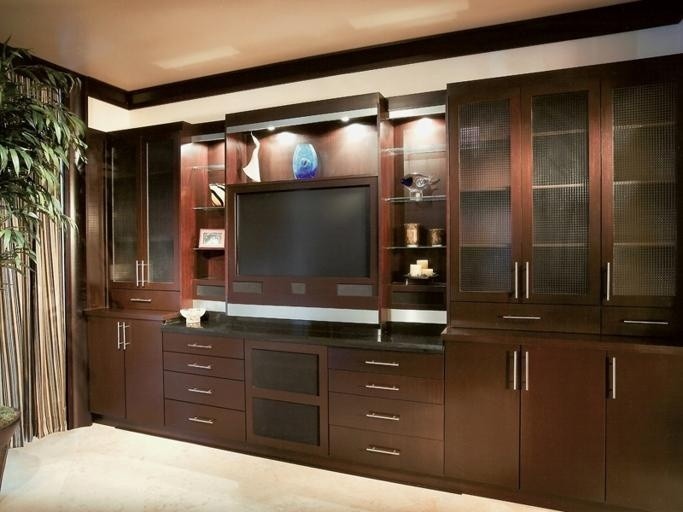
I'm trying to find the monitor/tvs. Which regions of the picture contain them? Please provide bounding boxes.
[225,176,379,311]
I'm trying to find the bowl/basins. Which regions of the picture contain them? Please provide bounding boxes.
[179,307,206,323]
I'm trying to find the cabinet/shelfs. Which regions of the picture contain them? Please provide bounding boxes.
[604,349,683,510]
[86,317,164,429]
[445,62,601,334]
[162,332,243,443]
[380,90,445,293]
[190,123,246,287]
[243,336,329,460]
[325,348,443,479]
[600,52,683,341]
[444,343,604,503]
[104,121,208,311]
